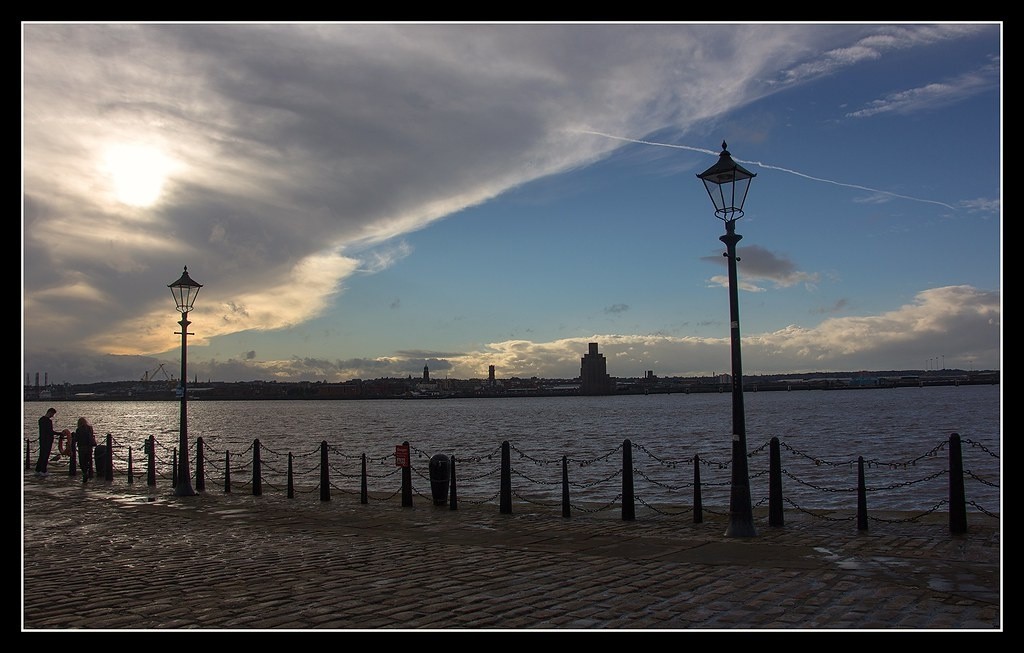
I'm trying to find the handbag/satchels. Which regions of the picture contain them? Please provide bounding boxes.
[90,435,97,446]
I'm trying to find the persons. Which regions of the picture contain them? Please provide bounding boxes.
[71,417,97,483]
[35,408,64,476]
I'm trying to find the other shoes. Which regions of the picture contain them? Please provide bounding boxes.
[40,471,48,477]
[34,470,41,476]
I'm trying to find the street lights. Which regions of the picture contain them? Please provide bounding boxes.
[694,140,760,540]
[166,265,203,499]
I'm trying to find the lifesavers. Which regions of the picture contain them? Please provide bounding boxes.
[58,430,73,456]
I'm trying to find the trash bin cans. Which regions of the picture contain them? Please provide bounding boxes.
[428,453,450,507]
[94,445,109,482]
